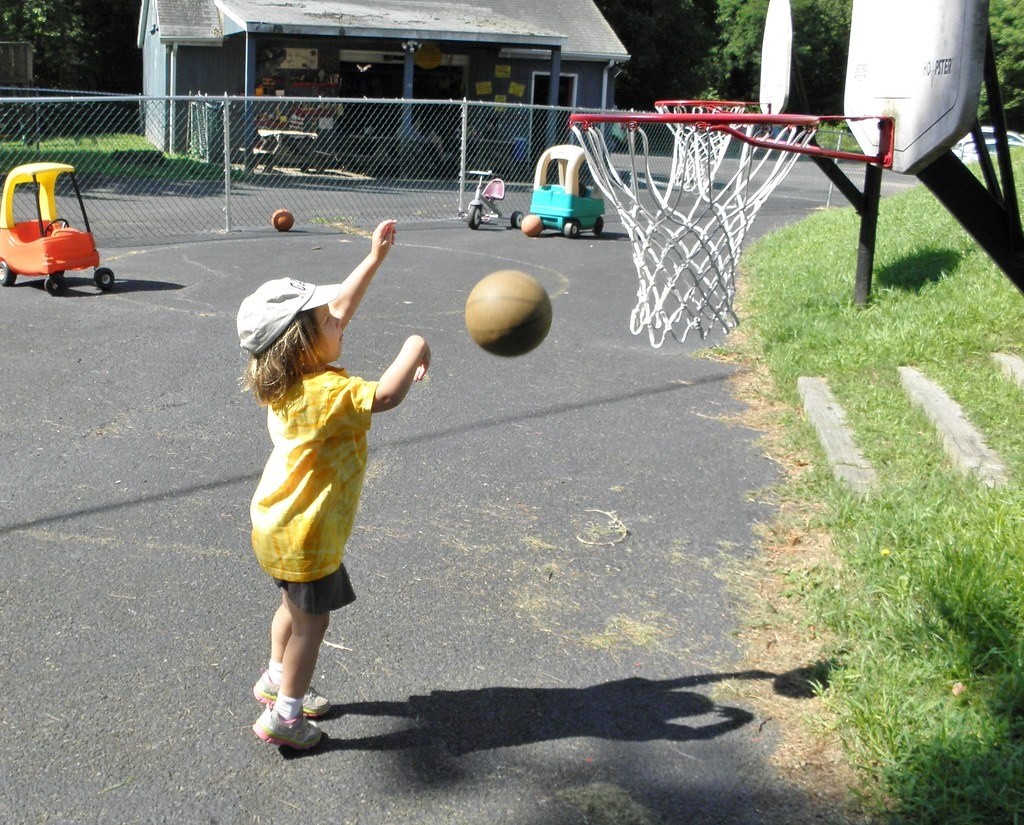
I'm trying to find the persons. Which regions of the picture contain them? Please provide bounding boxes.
[235,219,430,748]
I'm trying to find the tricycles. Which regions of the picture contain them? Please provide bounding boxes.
[468,170,524,230]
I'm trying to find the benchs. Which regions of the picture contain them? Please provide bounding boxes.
[239,148,274,173]
[309,149,348,173]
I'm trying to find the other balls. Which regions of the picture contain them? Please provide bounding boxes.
[520,214,544,236]
[271,208,294,232]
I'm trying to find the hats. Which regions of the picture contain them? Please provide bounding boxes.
[236,278,341,353]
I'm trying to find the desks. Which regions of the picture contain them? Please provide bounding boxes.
[351,136,415,179]
[458,136,517,180]
[258,129,318,173]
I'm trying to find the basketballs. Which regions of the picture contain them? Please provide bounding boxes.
[465,271,550,357]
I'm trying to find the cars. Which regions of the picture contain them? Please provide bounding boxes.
[531,144,607,238]
[4,163,116,297]
[954,124,1024,173]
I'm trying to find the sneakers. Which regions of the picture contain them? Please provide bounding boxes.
[253,702,321,750]
[253,668,330,717]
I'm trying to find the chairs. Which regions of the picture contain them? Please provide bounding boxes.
[482,178,505,201]
